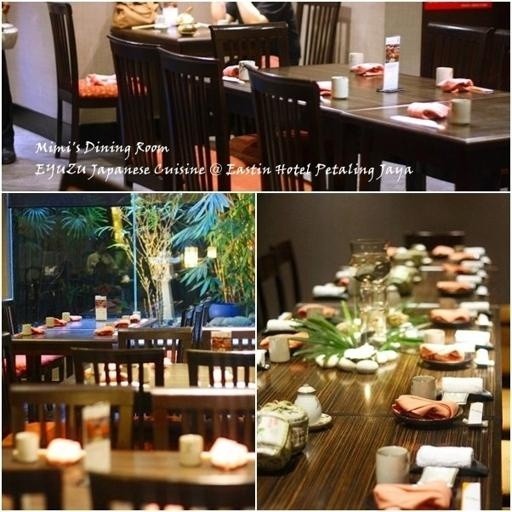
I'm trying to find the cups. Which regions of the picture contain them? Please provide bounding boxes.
[349,53,364,70]
[22,324,31,334]
[330,76,349,100]
[178,434,204,467]
[448,99,472,126]
[239,60,256,82]
[122,315,130,324]
[46,316,55,328]
[155,14,168,30]
[412,375,437,401]
[15,432,39,462]
[435,67,454,87]
[62,312,71,322]
[374,446,412,485]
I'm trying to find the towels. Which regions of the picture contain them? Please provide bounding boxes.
[257,240,475,510]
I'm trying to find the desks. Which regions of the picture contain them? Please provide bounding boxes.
[257,239,501,510]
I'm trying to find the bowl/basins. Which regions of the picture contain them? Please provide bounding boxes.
[208,302,241,321]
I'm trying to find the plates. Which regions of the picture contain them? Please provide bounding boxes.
[304,413,333,430]
[391,402,464,427]
[179,31,195,35]
[257,248,487,374]
[365,484,455,509]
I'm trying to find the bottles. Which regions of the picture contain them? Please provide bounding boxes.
[293,383,322,424]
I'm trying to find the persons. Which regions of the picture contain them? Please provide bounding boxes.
[2,2,16,164]
[211,2,301,66]
[86,240,115,275]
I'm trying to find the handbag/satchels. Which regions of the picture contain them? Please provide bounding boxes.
[111,2,162,26]
